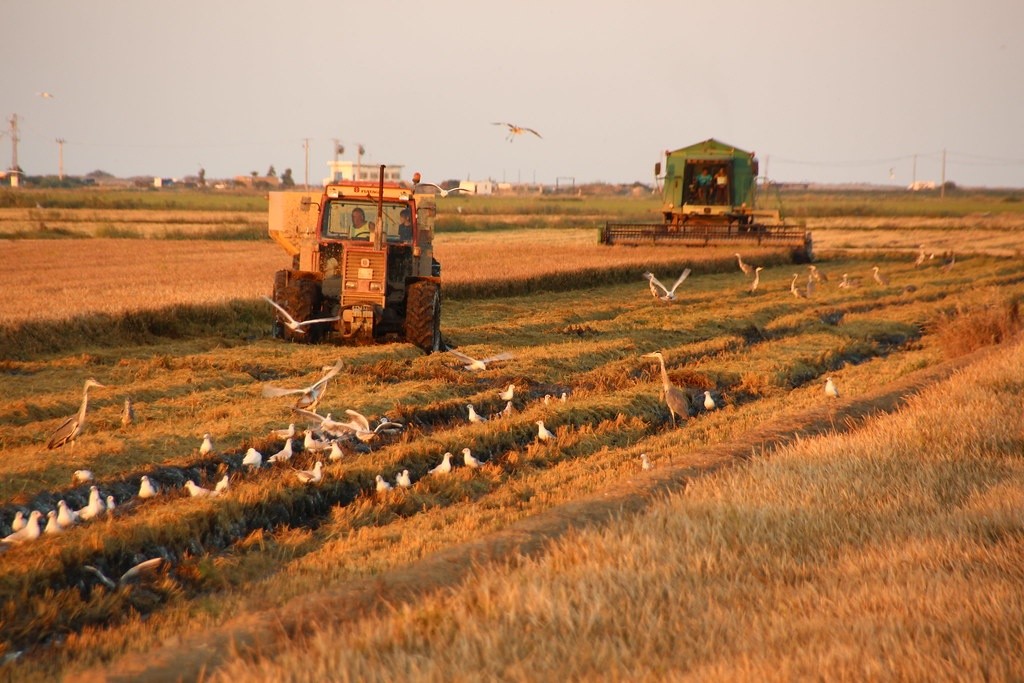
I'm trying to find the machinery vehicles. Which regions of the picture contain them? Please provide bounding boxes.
[267,161,443,356]
[597,137,813,248]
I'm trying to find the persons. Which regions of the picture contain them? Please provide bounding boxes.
[695,167,727,199]
[348,207,375,239]
[398,209,412,240]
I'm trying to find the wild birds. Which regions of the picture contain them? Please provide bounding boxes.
[704,390,716,412]
[535,420,557,443]
[643,352,691,430]
[493,121,542,143]
[427,452,454,475]
[76,558,164,609]
[0,469,115,545]
[46,376,134,449]
[445,345,513,372]
[467,384,566,424]
[642,244,936,307]
[639,453,652,472]
[184,358,412,499]
[138,475,156,498]
[261,294,341,334]
[825,377,841,402]
[461,447,485,469]
